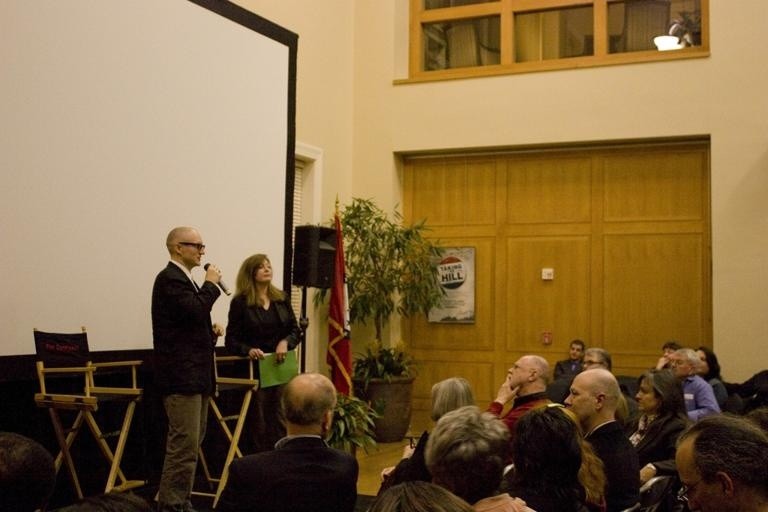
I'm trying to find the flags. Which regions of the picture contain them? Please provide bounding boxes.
[327,211,354,405]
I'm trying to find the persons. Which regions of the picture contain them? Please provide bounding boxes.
[65,493,151,508]
[377,378,473,497]
[485,354,554,495]
[564,369,640,509]
[499,403,608,508]
[224,254,301,452]
[627,368,693,487]
[546,340,641,439]
[152,226,225,510]
[3,431,55,509]
[670,347,722,430]
[677,413,766,509]
[424,405,533,509]
[368,481,474,508]
[655,342,729,408]
[215,371,359,508]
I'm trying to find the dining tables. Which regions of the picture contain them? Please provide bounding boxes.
[313,197,442,441]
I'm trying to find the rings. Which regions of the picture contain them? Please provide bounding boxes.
[409,438,412,449]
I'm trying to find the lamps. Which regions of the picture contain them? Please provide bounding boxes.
[497,374,746,512]
[33,321,150,496]
[177,348,259,512]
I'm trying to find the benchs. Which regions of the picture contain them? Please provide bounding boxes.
[327,211,354,405]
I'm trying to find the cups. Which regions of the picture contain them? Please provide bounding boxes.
[674,470,722,505]
[179,240,207,249]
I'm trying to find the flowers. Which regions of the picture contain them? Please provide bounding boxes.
[204,263,231,295]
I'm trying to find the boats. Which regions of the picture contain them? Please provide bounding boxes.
[293,226,336,288]
